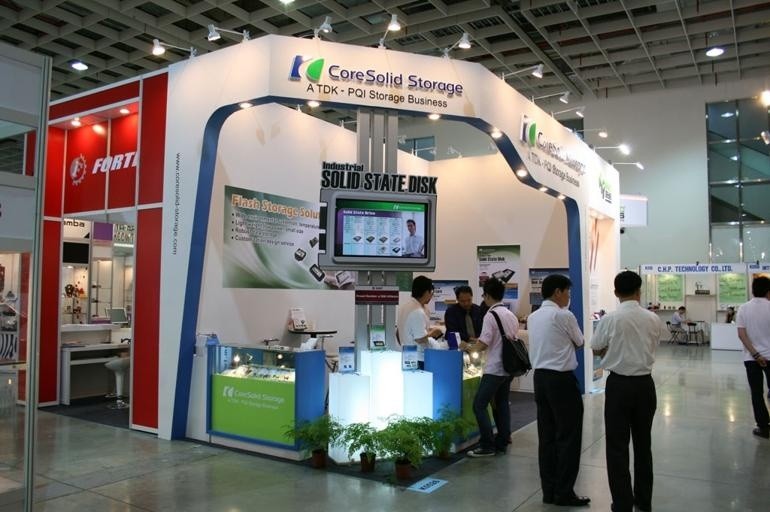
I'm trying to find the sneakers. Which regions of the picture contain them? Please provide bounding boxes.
[465,446,509,457]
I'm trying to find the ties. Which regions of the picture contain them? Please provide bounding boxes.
[465,311,476,340]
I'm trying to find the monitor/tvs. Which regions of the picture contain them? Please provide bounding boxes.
[61,240,90,267]
[334,198,428,258]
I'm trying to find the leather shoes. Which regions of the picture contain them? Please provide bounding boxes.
[753,428,770,439]
[542,491,591,507]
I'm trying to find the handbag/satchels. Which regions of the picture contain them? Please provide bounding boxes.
[489,310,532,378]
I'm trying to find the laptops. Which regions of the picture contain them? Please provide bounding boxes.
[105,307,129,324]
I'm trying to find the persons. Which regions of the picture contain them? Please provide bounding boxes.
[526,273,593,507]
[396,274,446,371]
[400,217,425,257]
[442,284,485,347]
[727,305,737,322]
[736,276,770,440]
[670,306,692,344]
[458,277,520,457]
[585,270,665,512]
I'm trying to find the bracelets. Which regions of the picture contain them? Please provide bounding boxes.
[752,351,761,361]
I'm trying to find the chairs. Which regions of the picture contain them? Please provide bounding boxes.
[665,320,705,347]
[104,358,130,409]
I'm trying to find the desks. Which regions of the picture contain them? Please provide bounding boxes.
[60,342,130,405]
[710,322,745,351]
